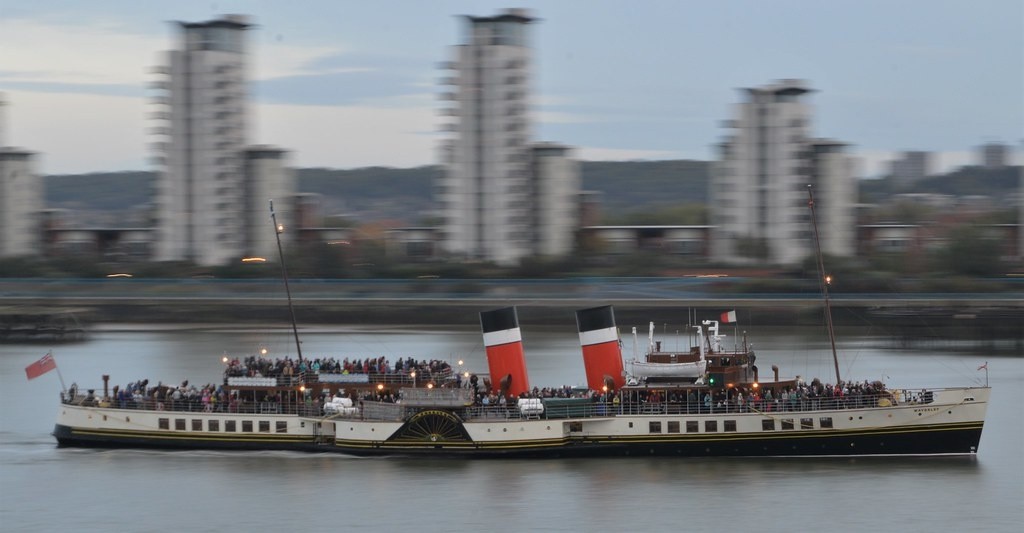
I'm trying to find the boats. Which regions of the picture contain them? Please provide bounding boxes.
[54,196,997,462]
[624,359,706,381]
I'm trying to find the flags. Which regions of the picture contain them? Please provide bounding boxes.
[25,353,57,380]
[720,310,736,323]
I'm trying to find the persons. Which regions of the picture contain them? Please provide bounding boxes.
[789,378,889,411]
[782,390,789,412]
[790,391,797,412]
[738,393,744,412]
[475,394,482,418]
[754,392,760,412]
[500,395,506,406]
[894,389,901,405]
[760,390,767,412]
[774,389,783,412]
[732,388,739,413]
[617,389,726,414]
[118,356,448,416]
[516,386,615,419]
[509,394,515,419]
[747,393,754,413]
[612,395,619,416]
[766,390,773,412]
[489,391,496,407]
[453,373,462,388]
[482,395,489,407]
[704,393,712,406]
[463,380,472,400]
[495,390,503,406]
